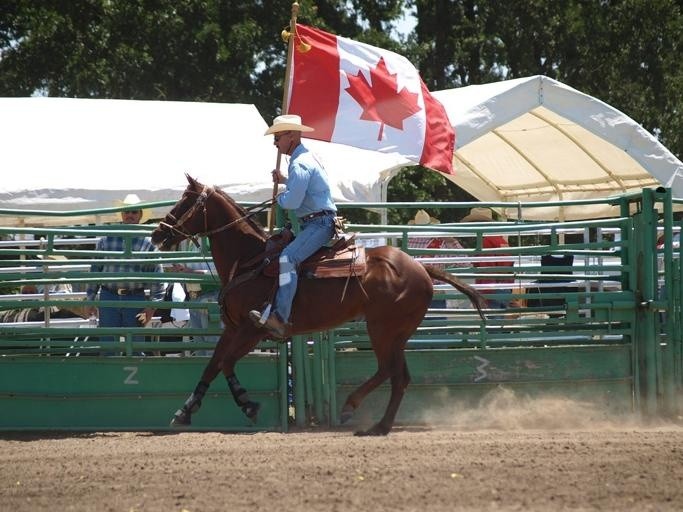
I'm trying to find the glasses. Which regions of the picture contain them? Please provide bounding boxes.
[274,131,292,142]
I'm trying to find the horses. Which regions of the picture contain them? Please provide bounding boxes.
[152,173,488,437]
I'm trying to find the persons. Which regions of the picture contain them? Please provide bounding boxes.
[150,280,189,355]
[247,114,338,338]
[84,194,163,357]
[34,248,71,296]
[405,208,474,349]
[459,207,516,334]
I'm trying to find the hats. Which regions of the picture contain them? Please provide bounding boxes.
[264,114,314,136]
[36,249,66,260]
[407,209,440,224]
[460,207,492,222]
[110,194,151,224]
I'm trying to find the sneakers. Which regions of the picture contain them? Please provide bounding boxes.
[248,310,286,338]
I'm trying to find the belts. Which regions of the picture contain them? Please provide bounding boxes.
[297,210,332,225]
[102,285,144,296]
[186,290,202,300]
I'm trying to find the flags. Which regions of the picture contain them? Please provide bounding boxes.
[280,24,455,174]
